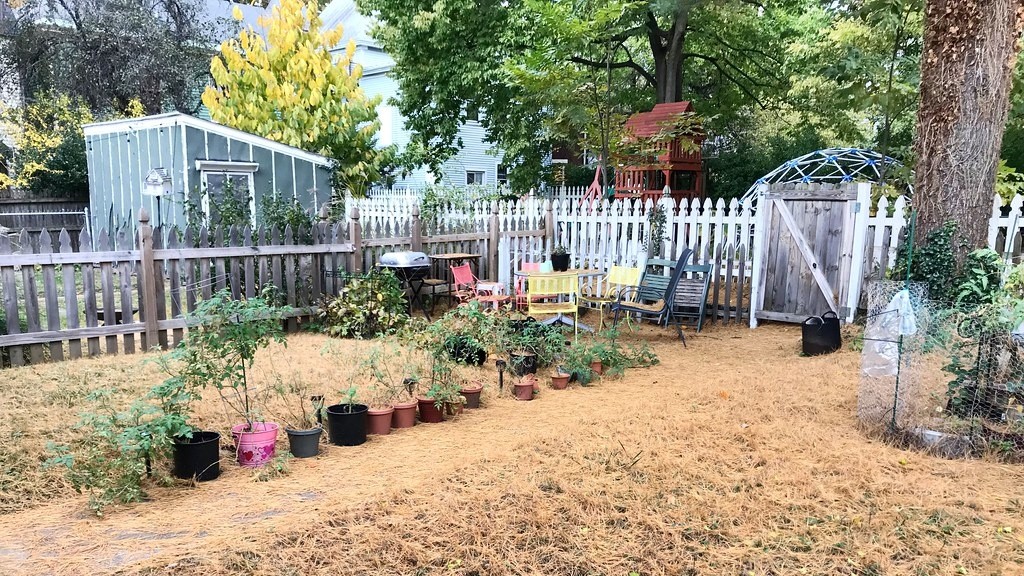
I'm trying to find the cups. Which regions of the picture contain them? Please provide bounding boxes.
[539,259,554,274]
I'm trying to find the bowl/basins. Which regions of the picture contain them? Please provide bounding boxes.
[521,262,539,274]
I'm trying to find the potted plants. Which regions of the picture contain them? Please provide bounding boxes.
[551,243,571,273]
[61,277,659,517]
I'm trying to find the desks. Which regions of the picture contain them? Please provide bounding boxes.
[429,253,483,314]
[514,268,606,334]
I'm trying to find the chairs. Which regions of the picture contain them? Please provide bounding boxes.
[516,261,557,315]
[664,263,713,331]
[526,271,579,344]
[450,261,510,320]
[629,255,680,326]
[578,265,641,332]
[611,248,693,347]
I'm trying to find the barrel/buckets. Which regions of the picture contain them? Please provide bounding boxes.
[801,310,842,357]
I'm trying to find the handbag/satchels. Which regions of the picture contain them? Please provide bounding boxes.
[802,311,842,357]
